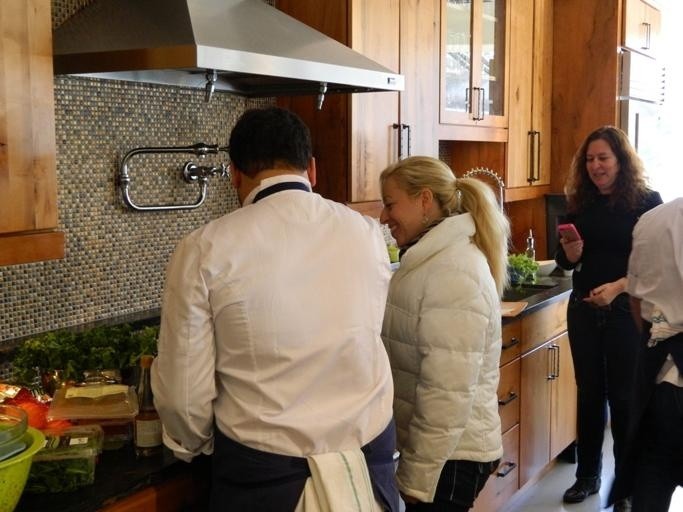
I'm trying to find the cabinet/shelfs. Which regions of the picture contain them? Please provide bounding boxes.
[545,0,667,196]
[474,298,577,512]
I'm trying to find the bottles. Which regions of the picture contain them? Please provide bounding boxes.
[525,228,536,261]
[130,354,163,458]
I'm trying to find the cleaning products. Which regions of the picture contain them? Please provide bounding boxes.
[524,228,536,281]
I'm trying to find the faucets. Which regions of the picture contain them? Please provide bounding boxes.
[455,166,506,215]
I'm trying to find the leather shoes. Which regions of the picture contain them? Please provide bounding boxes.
[563,477,600,504]
[613,495,633,512]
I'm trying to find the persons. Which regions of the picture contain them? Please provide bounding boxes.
[150,106,409,511]
[554,127,662,511]
[625,195,682,511]
[376,156,507,512]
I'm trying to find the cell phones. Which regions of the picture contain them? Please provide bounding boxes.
[557,223,581,242]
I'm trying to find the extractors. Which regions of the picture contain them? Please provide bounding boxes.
[53,0,404,109]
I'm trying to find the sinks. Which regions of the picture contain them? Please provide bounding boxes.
[499,282,559,303]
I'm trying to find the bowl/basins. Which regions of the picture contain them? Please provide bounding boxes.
[533,259,559,277]
